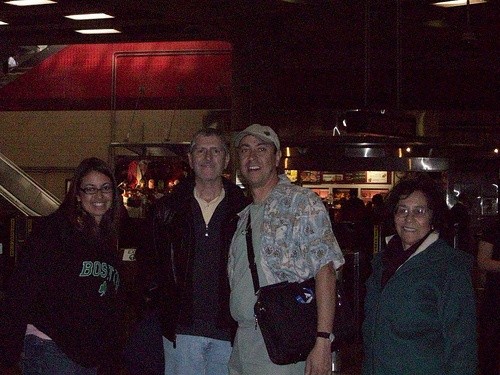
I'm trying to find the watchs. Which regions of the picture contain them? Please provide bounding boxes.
[316,332,335,342]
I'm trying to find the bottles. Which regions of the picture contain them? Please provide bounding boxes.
[127,189,142,208]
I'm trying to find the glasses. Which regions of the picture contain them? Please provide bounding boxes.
[79,185,113,195]
[394,205,431,218]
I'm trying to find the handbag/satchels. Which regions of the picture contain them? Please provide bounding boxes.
[253,277,360,366]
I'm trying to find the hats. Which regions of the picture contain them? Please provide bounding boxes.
[233,124,280,152]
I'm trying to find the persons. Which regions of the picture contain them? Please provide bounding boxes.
[478,219,500,375]
[227,123,346,375]
[144,128,253,375]
[18,158,125,375]
[347,189,384,213]
[360,175,478,375]
[452,192,469,234]
